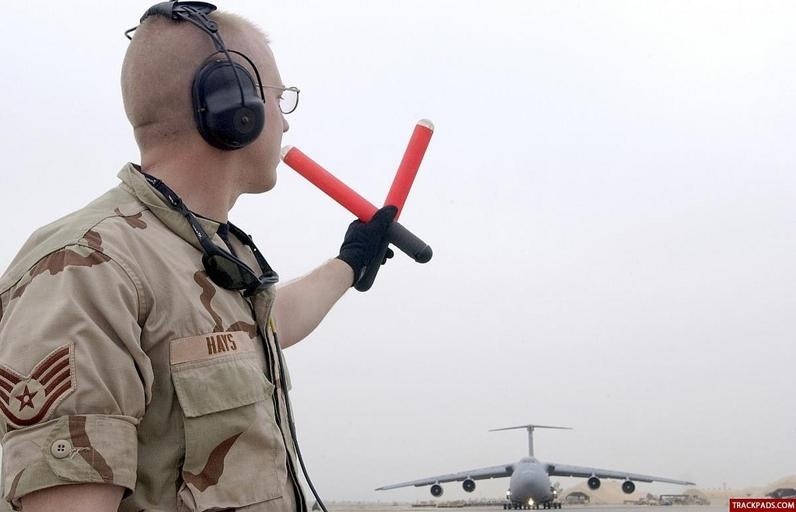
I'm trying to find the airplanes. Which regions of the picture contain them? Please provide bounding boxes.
[375,424,695,510]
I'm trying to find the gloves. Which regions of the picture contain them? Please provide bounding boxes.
[336,204,398,291]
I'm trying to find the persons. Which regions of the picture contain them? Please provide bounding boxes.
[0,0,398,512]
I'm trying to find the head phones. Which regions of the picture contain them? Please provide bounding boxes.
[125,0,267,151]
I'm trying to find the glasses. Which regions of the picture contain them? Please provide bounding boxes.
[248,84,300,114]
[139,171,279,297]
